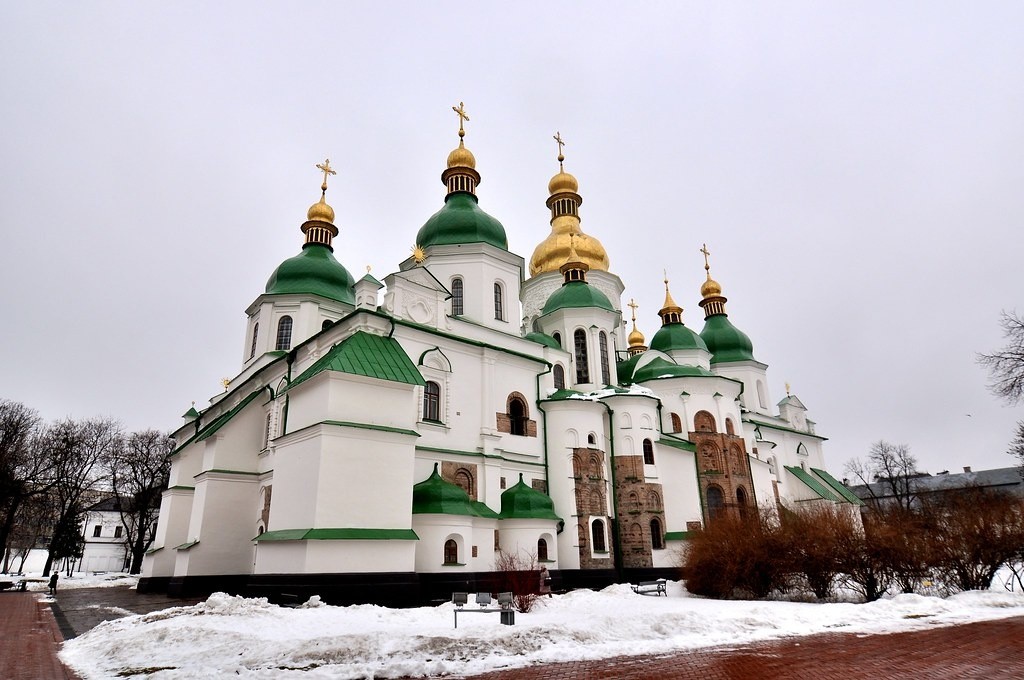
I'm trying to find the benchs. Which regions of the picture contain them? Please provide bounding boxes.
[92,571,107,576]
[10,572,26,577]
[631,580,667,597]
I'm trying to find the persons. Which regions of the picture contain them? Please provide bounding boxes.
[540,565,552,598]
[50,571,58,595]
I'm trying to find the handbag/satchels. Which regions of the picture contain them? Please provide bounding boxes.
[544,576,551,587]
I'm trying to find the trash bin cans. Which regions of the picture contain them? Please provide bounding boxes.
[501,610,515,625]
